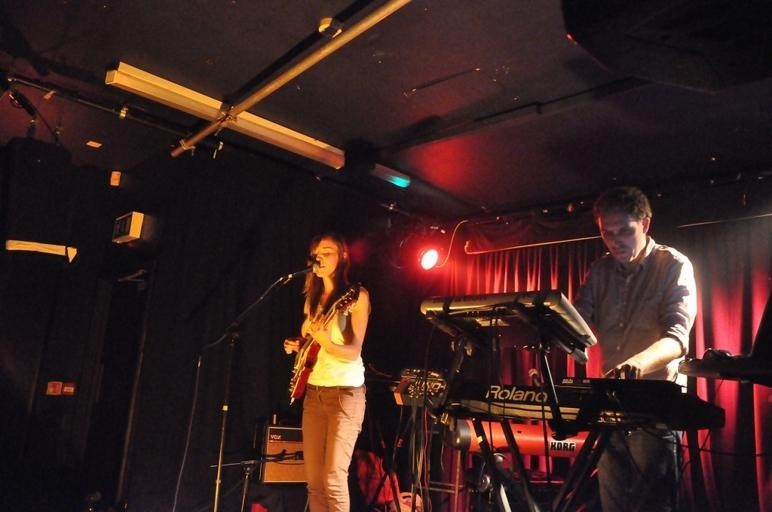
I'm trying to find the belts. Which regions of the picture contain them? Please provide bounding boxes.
[305,385,363,391]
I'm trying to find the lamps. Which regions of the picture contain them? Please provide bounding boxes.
[396,223,440,271]
[105,58,344,173]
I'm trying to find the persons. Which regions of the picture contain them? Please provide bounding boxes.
[575,187,698,512]
[284,232,370,512]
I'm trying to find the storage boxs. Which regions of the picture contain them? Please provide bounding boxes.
[259,427,305,483]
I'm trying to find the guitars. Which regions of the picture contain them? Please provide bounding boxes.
[288,282,360,406]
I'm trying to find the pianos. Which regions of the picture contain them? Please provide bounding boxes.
[392,375,725,434]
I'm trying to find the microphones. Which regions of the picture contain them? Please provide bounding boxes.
[527,368,544,390]
[287,263,320,280]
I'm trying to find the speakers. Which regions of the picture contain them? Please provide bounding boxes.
[256,424,318,489]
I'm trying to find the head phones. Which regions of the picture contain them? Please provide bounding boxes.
[475,454,514,493]
